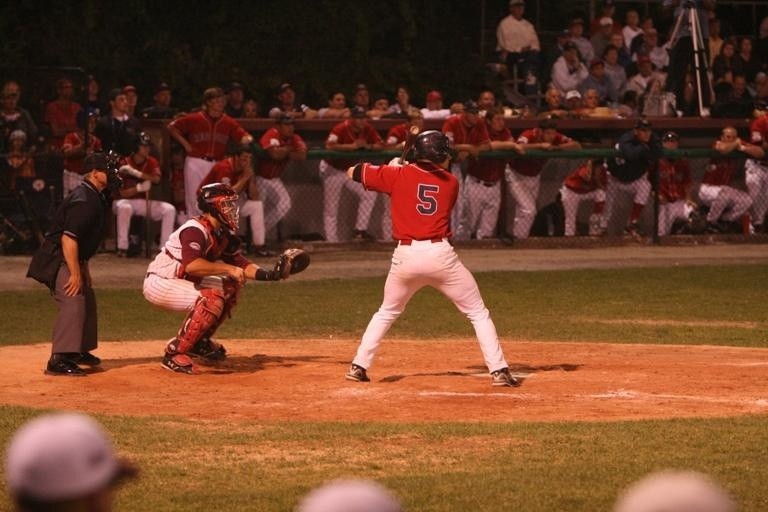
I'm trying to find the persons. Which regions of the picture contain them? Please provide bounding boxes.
[1,65,768,259]
[142,185,286,375]
[22,148,118,378]
[492,0,767,117]
[344,128,522,386]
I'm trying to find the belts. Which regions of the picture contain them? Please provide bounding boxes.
[754,160,768,167]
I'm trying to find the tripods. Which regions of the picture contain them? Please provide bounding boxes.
[656,9,716,117]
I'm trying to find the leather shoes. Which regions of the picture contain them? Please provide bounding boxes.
[161,350,193,375]
[45,353,87,376]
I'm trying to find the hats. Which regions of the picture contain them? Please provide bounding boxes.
[201,78,504,125]
[506,1,678,142]
[83,152,108,174]
[3,72,171,147]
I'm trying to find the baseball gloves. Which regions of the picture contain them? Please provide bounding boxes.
[267,249,310,282]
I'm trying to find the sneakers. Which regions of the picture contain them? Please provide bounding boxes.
[345,364,371,383]
[490,368,521,387]
[622,223,649,237]
[185,338,226,363]
[118,250,126,257]
[707,223,723,233]
[351,229,373,243]
[68,351,100,366]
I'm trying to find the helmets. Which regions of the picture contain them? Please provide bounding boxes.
[414,129,450,163]
[197,182,240,232]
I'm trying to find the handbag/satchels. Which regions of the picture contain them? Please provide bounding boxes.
[26,238,63,290]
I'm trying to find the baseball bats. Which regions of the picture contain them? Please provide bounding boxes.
[144,190,154,258]
[399,125,420,164]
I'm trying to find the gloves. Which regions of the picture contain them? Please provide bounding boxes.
[136,180,152,193]
[119,164,144,179]
